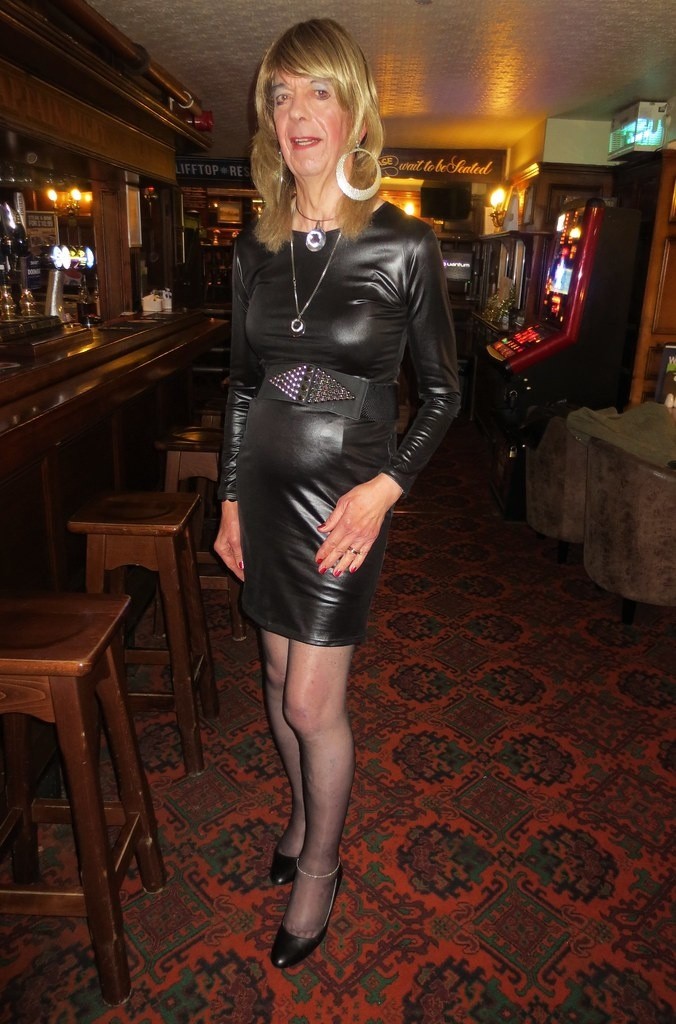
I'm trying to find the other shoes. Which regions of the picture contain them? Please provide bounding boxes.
[270,851,299,885]
[271,865,346,967]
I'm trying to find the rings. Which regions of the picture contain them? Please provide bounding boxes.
[348,546,359,555]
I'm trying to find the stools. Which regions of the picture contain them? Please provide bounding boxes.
[157,426,248,642]
[0,593,168,1008]
[68,491,220,778]
[192,398,227,552]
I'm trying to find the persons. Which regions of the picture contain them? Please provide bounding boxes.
[213,19,464,972]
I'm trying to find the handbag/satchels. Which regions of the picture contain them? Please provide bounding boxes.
[519,402,578,448]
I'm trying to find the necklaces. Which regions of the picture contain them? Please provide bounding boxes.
[285,214,348,338]
[293,191,341,253]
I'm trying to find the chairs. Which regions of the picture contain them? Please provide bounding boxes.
[521,396,589,544]
[584,403,676,608]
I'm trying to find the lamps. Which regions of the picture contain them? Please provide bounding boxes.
[47,186,83,218]
